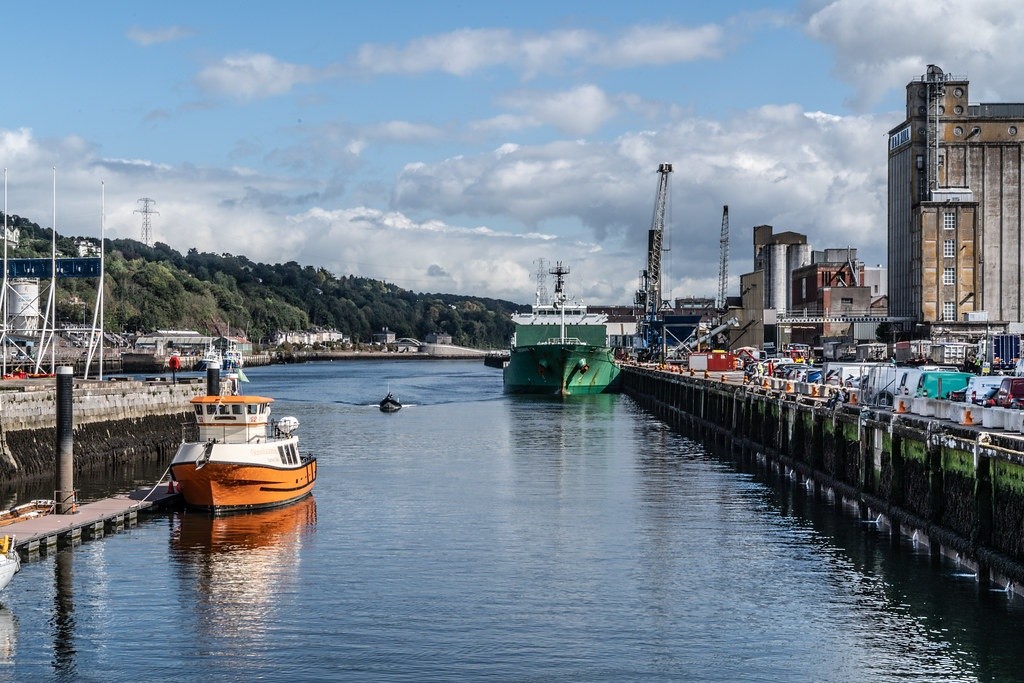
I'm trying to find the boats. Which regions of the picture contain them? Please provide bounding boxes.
[171,395,317,516]
[0,499,55,527]
[0,535,22,593]
[380,391,403,410]
[503,259,621,398]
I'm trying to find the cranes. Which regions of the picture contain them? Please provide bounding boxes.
[632,159,739,363]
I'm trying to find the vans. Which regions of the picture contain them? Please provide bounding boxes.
[751,357,1024,410]
